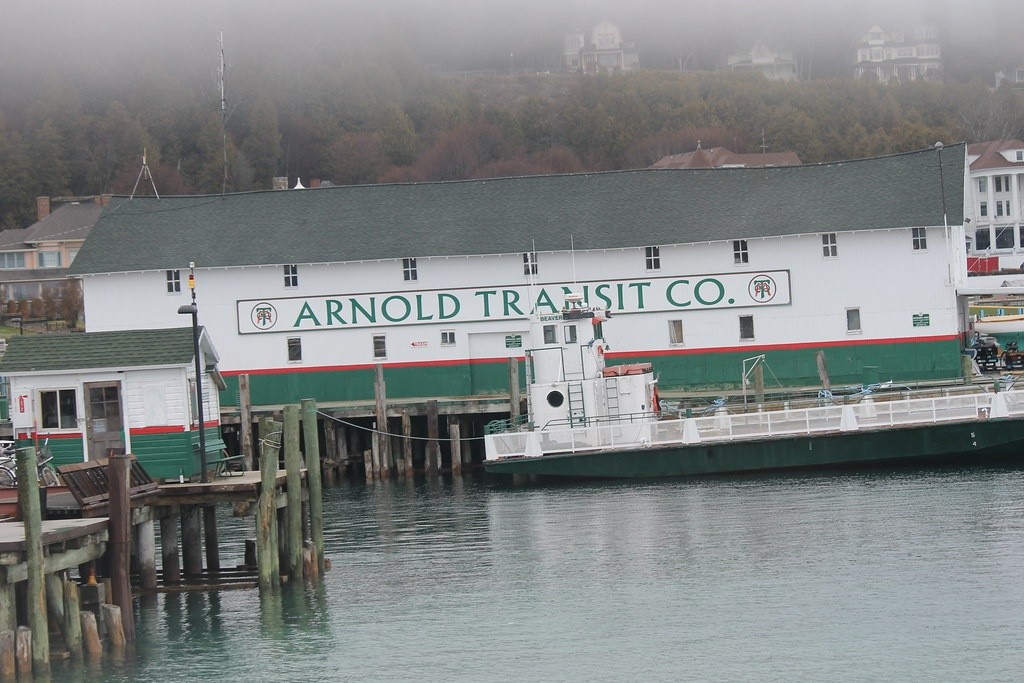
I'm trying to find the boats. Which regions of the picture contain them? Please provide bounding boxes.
[481,285,1024,478]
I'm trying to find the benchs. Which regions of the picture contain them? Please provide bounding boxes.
[192,440,246,480]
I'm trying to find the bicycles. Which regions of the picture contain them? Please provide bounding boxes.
[0,432,63,491]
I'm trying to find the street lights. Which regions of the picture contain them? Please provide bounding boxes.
[10,315,24,337]
[178,304,211,483]
